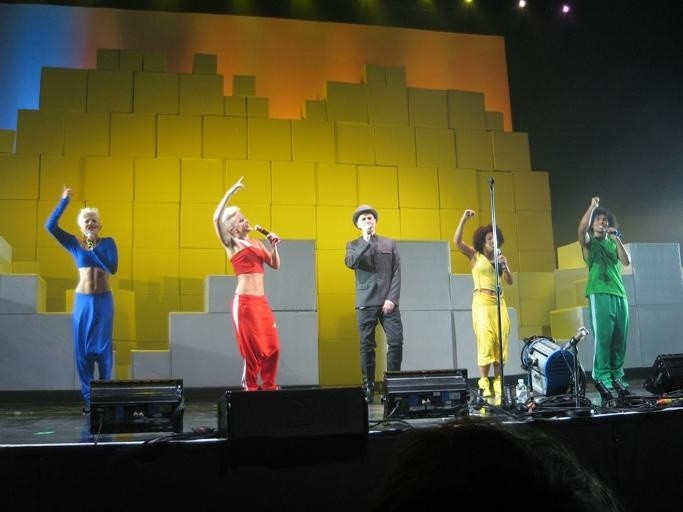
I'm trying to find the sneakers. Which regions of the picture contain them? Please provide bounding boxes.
[478,377,491,397]
[603,379,613,390]
[493,380,502,396]
[617,380,629,388]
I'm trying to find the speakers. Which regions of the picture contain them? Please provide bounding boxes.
[218,388,368,439]
[644,353,683,395]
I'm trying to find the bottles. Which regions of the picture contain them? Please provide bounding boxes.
[504,384,513,407]
[515,378,527,404]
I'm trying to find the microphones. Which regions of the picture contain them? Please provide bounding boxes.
[87,231,94,248]
[603,226,624,237]
[255,225,275,241]
[497,249,507,272]
[367,222,372,234]
[564,326,590,349]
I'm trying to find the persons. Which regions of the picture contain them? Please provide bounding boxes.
[453,209,512,396]
[578,196,631,393]
[345,206,403,402]
[213,176,281,392]
[46,184,118,417]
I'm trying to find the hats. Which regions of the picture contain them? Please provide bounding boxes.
[353,206,378,230]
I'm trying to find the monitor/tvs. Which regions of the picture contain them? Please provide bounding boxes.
[90,380,185,433]
[384,369,467,418]
